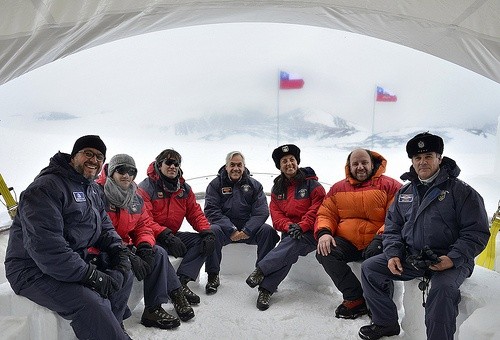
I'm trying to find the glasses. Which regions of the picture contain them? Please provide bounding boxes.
[78,150,104,161]
[160,158,179,167]
[114,166,135,176]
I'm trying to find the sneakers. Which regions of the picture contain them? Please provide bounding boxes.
[169,288,194,322]
[335,296,368,318]
[141,306,180,329]
[257,287,273,310]
[246,265,264,288]
[179,279,200,304]
[358,321,400,340]
[206,273,220,294]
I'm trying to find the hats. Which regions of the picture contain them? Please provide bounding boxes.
[108,154,138,181]
[71,135,107,164]
[272,144,300,169]
[406,131,443,159]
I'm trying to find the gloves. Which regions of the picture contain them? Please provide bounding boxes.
[287,223,302,239]
[107,245,131,288]
[157,228,187,258]
[200,231,216,257]
[83,265,119,298]
[406,245,449,278]
[134,243,154,271]
[127,250,151,281]
[367,235,383,256]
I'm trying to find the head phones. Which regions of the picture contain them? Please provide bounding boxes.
[419,268,432,290]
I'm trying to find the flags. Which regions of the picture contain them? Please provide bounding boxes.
[376,86,397,102]
[280,71,304,89]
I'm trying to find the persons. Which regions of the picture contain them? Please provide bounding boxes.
[204,151,280,295]
[136,149,211,323]
[86,154,181,329]
[314,148,404,319]
[245,145,325,311]
[3,135,133,340]
[358,132,491,340]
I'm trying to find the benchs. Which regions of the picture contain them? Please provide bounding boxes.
[0,192,500,340]
